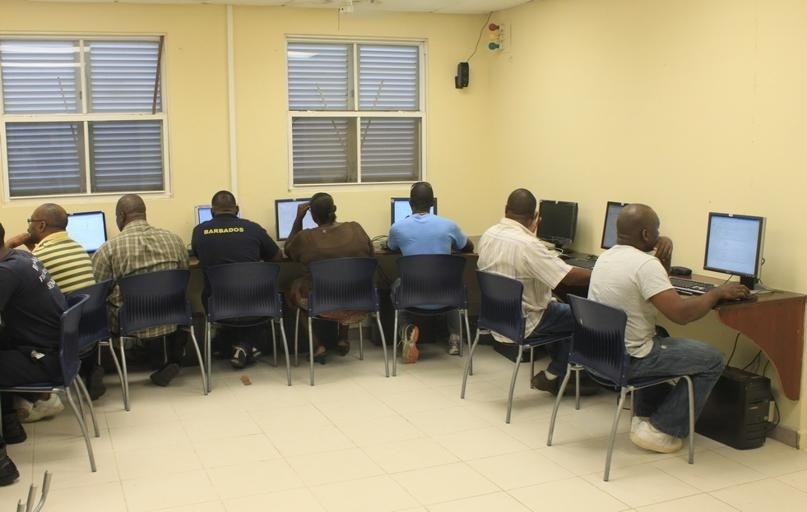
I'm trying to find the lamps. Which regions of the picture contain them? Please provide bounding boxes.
[486,21,506,53]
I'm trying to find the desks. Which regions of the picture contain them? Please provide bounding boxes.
[558,244,807,405]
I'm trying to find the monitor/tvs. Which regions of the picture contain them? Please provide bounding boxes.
[276,198,320,241]
[65,211,107,254]
[535,200,578,253]
[390,198,437,227]
[600,201,630,249]
[194,204,213,227]
[703,212,766,288]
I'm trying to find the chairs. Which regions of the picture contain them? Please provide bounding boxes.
[294,257,389,385]
[460,270,579,423]
[0,293,100,473]
[98,268,208,411]
[64,279,127,431]
[205,262,292,392]
[392,254,474,377]
[547,294,694,482]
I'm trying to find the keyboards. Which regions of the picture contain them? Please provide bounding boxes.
[668,275,717,296]
[564,258,596,270]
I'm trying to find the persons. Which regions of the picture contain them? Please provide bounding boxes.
[191,190,283,368]
[385,181,474,363]
[91,193,190,386]
[12,203,96,423]
[284,191,376,365]
[581,204,750,453]
[476,188,598,396]
[0,223,72,487]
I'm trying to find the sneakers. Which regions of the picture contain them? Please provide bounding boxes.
[305,346,328,362]
[0,445,21,487]
[630,415,684,455]
[334,338,350,357]
[560,377,602,397]
[447,335,461,355]
[0,392,66,445]
[245,346,262,368]
[398,323,421,365]
[85,363,107,403]
[150,362,181,387]
[530,370,567,398]
[230,344,247,370]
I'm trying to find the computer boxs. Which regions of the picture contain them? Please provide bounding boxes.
[693,365,770,449]
[494,340,536,363]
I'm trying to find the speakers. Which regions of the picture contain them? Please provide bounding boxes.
[458,62,469,88]
